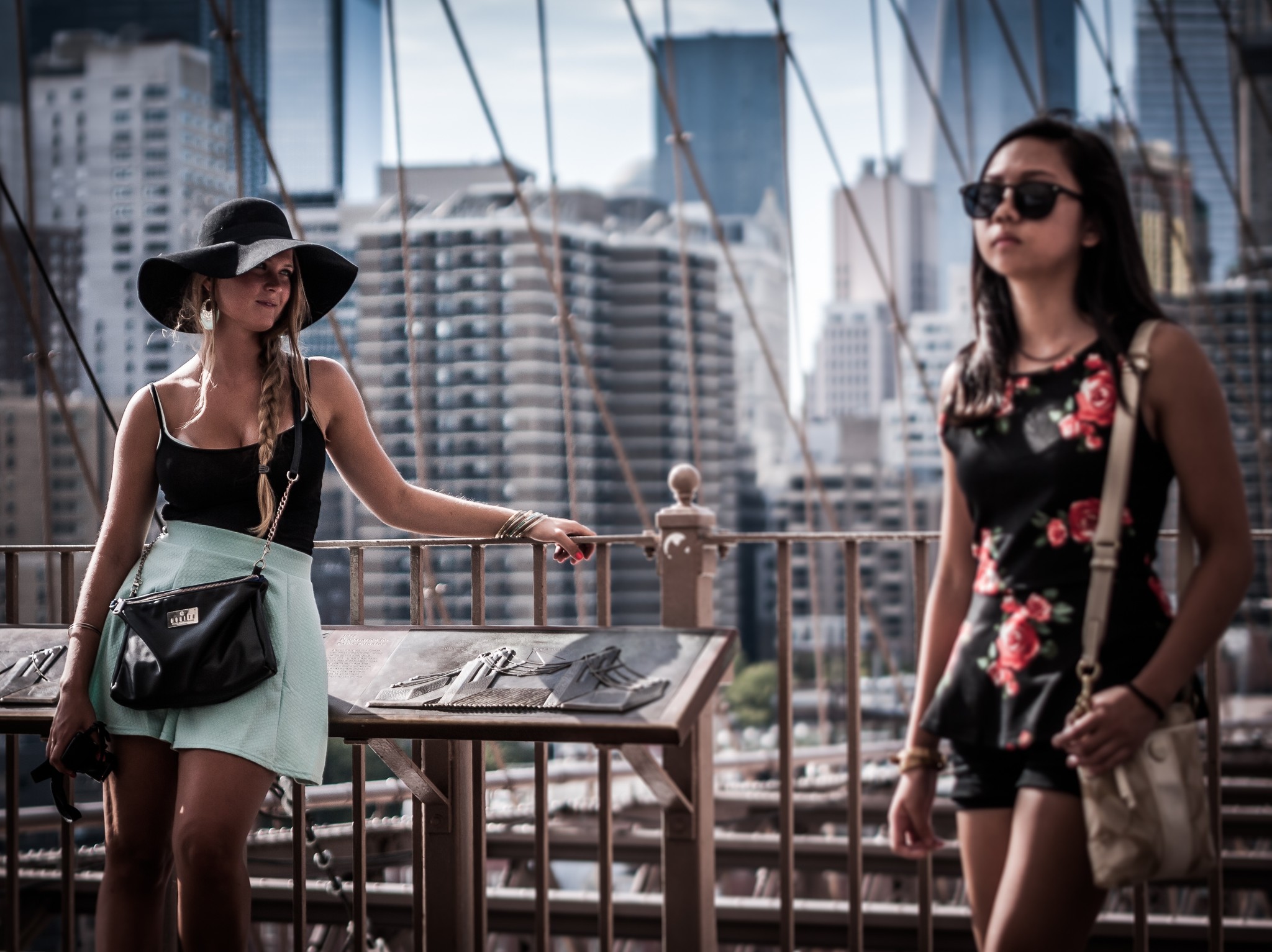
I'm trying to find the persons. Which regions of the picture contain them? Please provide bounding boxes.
[47,196,600,952]
[885,116,1255,952]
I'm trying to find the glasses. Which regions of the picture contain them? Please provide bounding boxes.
[960,181,1080,220]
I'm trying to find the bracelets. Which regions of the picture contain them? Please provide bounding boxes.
[1124,680,1164,721]
[889,746,948,773]
[494,509,548,539]
[65,622,101,639]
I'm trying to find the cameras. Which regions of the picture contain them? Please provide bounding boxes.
[59,730,119,783]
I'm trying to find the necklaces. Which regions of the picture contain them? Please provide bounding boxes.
[1018,339,1080,361]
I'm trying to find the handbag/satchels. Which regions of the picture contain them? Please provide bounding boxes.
[109,575,276,711]
[1077,718,1214,888]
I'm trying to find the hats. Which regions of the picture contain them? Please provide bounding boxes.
[136,198,359,334]
